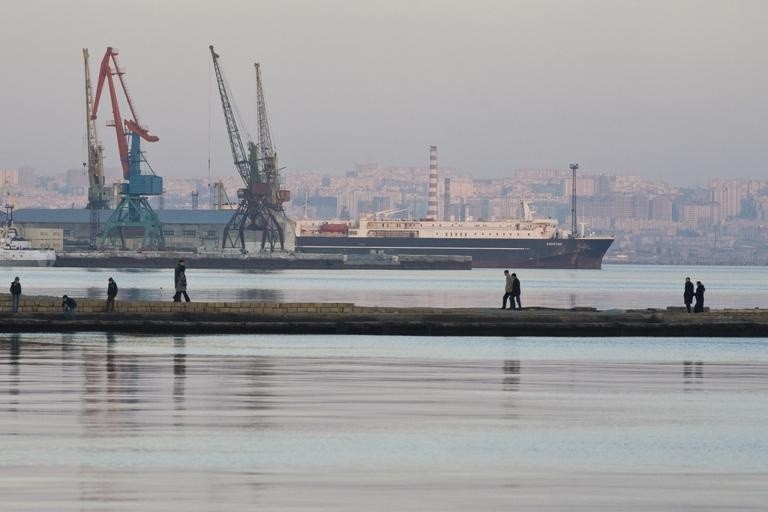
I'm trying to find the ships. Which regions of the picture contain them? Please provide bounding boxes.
[293,216,616,270]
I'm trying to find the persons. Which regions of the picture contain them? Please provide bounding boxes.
[61,294,78,312]
[693,281,705,313]
[103,277,118,312]
[173,266,191,302]
[508,273,522,311]
[9,277,21,313]
[683,276,694,314]
[500,269,515,309]
[171,259,186,302]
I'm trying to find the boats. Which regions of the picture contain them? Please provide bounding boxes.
[0,218,56,268]
